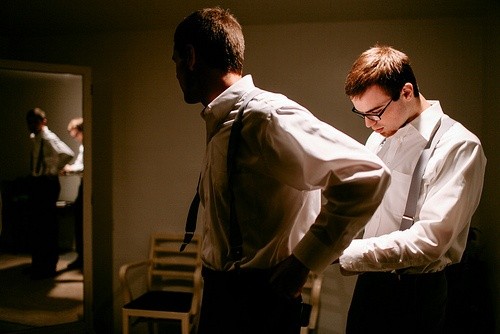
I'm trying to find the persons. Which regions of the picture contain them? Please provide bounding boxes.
[169,8,392,334]
[58,118,85,270]
[330,47,487,334]
[24,104,74,281]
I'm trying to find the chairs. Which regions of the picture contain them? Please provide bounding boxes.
[119,231,204,334]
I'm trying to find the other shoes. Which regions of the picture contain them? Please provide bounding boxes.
[68,255,82,270]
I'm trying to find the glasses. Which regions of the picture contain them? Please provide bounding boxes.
[350,98,392,122]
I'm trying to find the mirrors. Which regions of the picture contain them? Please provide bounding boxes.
[0,62,95,334]
